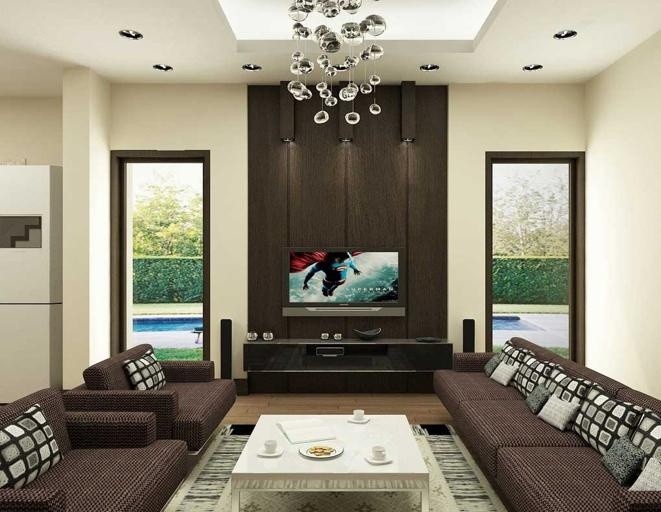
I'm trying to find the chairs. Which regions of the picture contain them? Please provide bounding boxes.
[58,341,238,458]
[1,384,195,512]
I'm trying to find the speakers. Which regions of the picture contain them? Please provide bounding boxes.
[463,319,475,352]
[221,319,231,379]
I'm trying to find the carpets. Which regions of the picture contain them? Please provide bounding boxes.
[159,422,512,512]
[213,422,460,511]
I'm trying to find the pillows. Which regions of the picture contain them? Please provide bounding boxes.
[1,401,68,493]
[121,348,170,393]
[479,336,660,493]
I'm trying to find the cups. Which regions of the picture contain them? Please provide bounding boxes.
[262,332,275,341]
[247,333,261,341]
[334,334,343,341]
[264,438,279,452]
[372,445,386,460]
[321,333,330,339]
[354,408,365,420]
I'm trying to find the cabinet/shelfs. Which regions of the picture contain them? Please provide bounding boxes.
[243,336,456,395]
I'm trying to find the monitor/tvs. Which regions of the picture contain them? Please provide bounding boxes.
[280,246,407,317]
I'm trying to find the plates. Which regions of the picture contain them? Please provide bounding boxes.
[256,447,286,457]
[349,419,373,423]
[366,455,393,466]
[417,338,443,342]
[353,327,382,340]
[298,443,346,458]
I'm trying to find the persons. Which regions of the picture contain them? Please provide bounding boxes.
[303,252,362,296]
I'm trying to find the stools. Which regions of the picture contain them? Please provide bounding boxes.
[189,324,204,345]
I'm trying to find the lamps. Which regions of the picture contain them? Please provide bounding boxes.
[277,77,298,144]
[281,0,387,129]
[395,80,419,144]
[333,78,356,144]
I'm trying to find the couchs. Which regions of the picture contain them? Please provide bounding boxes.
[429,332,660,511]
